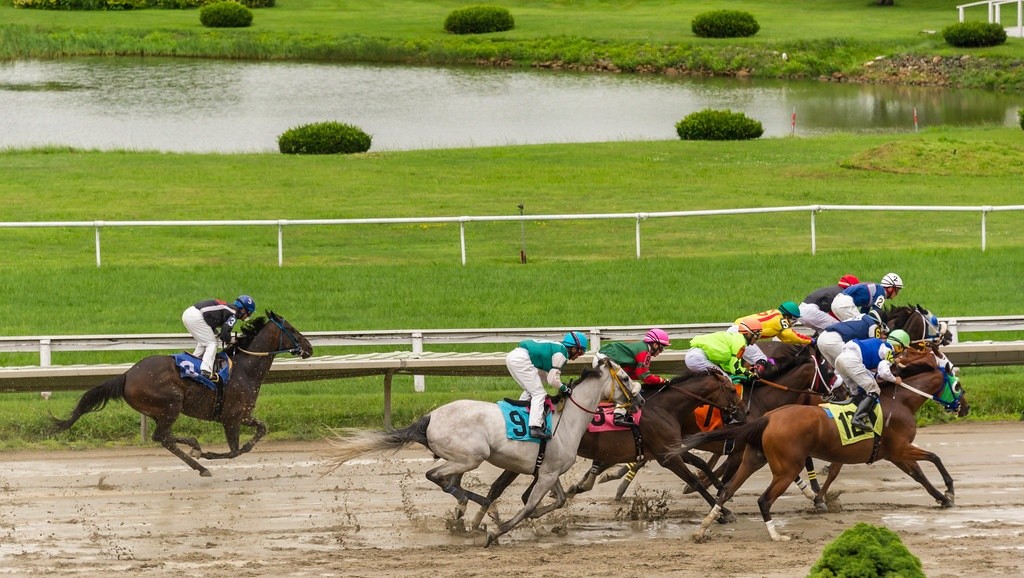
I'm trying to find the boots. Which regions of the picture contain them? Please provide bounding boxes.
[851,397,875,430]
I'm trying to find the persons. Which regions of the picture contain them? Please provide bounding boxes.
[181,295,255,380]
[684,318,763,383]
[505,331,588,439]
[817,308,890,395]
[726,301,816,365]
[831,272,904,322]
[834,330,910,430]
[796,274,860,330]
[591,329,671,428]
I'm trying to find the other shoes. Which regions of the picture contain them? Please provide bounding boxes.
[530,427,551,438]
[201,371,217,381]
[613,414,633,426]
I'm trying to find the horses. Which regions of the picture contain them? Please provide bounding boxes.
[312,302,970,548]
[49,308,314,477]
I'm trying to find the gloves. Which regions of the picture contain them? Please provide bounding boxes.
[812,338,816,344]
[561,385,572,394]
[229,336,239,345]
[214,328,220,335]
[664,378,671,383]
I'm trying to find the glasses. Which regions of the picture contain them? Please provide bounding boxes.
[790,320,798,325]
[658,346,664,352]
[575,347,585,357]
[754,332,761,338]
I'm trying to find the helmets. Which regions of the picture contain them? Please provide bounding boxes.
[643,329,671,346]
[779,301,801,318]
[738,317,763,334]
[562,331,588,351]
[868,309,887,324]
[889,329,911,348]
[236,296,256,315]
[881,273,906,289]
[838,275,859,288]
